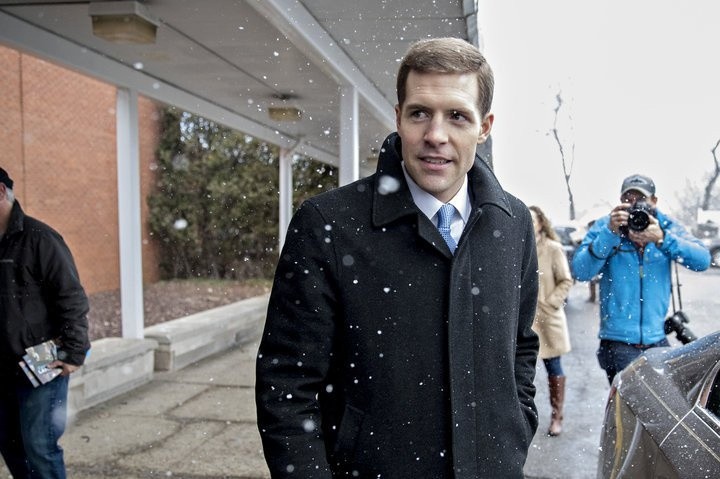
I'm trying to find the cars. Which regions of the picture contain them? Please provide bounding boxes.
[694,221,720,268]
[550,222,588,260]
[596,330,720,479]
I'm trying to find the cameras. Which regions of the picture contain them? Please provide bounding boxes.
[617,204,657,236]
[662,311,697,345]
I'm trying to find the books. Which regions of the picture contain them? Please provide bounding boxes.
[19,340,62,387]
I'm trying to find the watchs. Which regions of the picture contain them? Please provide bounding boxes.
[656,239,664,247]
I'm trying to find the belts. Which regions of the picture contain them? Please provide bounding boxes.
[629,343,656,350]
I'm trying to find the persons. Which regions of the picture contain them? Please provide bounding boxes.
[528,205,573,437]
[568,173,711,387]
[0,166,91,479]
[577,220,597,303]
[255,36,540,479]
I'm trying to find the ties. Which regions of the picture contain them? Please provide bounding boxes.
[437,203,459,255]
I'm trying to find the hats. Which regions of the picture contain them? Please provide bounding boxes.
[619,176,655,198]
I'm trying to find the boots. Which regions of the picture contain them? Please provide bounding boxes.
[549,375,566,436]
